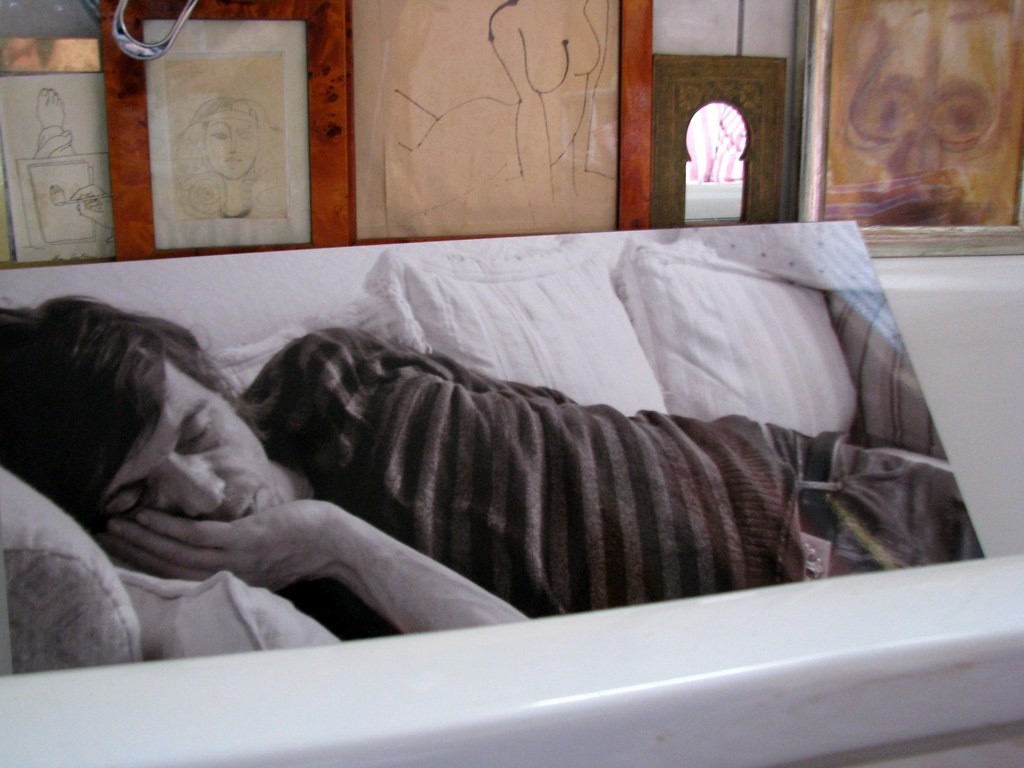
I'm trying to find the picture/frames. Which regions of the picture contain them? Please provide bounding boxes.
[99,0,355,262]
[652,53,795,230]
[787,1,1024,258]
[0,35,116,269]
[347,0,654,249]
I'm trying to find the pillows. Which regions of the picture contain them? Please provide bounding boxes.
[112,564,344,664]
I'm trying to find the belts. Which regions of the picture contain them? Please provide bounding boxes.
[799,427,847,581]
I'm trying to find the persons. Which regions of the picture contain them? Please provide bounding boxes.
[1,294,988,645]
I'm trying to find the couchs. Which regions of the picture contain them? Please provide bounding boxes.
[0,221,1024,679]
